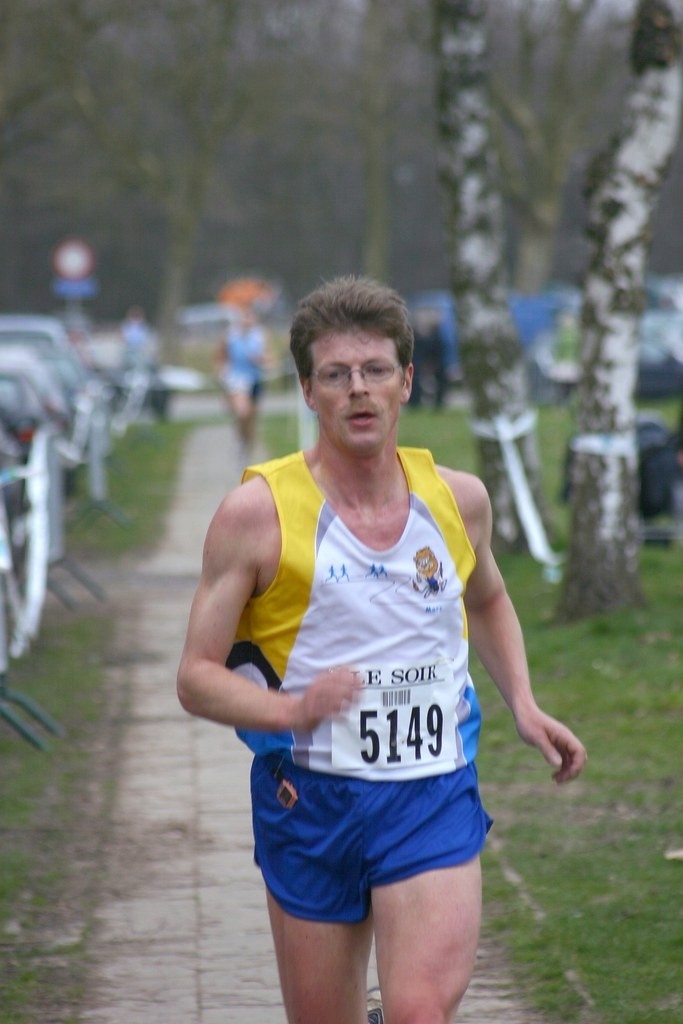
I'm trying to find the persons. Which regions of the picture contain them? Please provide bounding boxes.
[214,306,268,457]
[175,275,588,1023]
[555,308,581,402]
[401,309,448,412]
[120,306,166,421]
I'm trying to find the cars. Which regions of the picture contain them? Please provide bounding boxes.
[641,281,683,395]
[0,310,108,567]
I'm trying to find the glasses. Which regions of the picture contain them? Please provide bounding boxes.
[303,361,402,388]
